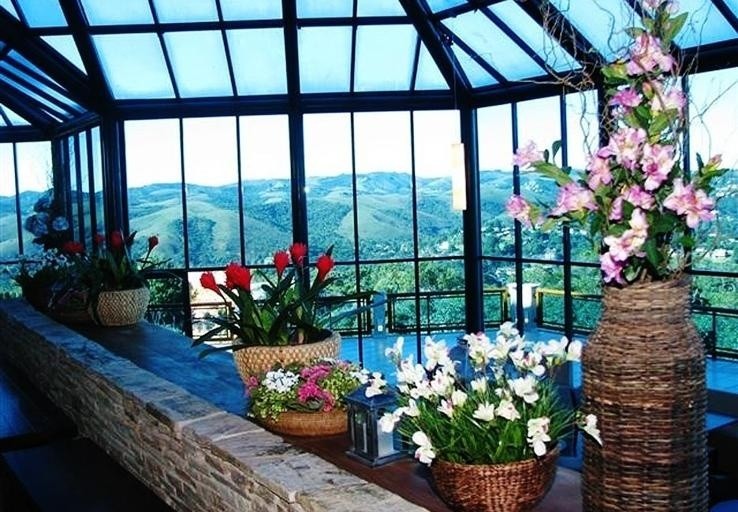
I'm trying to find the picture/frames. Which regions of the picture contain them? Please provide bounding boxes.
[190,301,233,343]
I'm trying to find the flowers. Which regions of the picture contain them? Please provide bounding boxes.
[22,185,185,310]
[352,320,603,465]
[504,0,727,284]
[242,358,359,420]
[191,243,350,348]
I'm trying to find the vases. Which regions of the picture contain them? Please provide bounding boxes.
[256,410,348,436]
[84,285,151,330]
[425,443,566,512]
[578,284,712,511]
[237,328,342,385]
